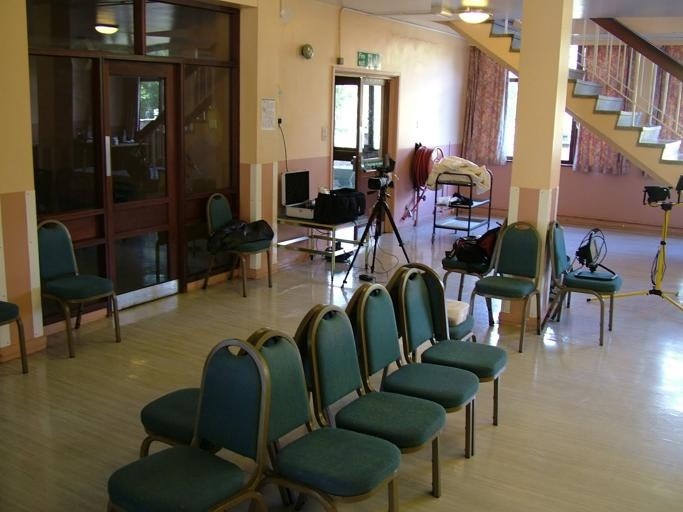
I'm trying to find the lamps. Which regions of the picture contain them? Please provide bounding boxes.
[94,23,120,36]
[456,0,493,23]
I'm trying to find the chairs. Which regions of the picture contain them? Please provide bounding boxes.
[107,337,272,510]
[357,284,480,499]
[0,299,30,373]
[307,306,448,511]
[139,327,271,454]
[400,262,477,343]
[468,220,541,353]
[249,330,401,510]
[36,218,122,358]
[202,193,274,298]
[398,267,507,456]
[289,262,413,404]
[442,217,507,327]
[541,220,623,346]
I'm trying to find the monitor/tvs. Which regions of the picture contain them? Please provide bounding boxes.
[281,170,310,206]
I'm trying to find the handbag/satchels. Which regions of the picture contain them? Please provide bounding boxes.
[314,188,365,225]
[453,227,500,274]
[207,220,274,253]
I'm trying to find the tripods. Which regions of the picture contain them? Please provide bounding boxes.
[587,210,683,312]
[341,188,410,288]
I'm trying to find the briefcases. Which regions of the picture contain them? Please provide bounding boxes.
[280,169,316,218]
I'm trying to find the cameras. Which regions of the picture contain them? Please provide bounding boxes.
[367,158,396,190]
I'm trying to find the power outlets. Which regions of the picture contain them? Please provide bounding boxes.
[276,116,286,126]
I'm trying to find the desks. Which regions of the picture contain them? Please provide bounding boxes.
[275,216,378,283]
[111,142,153,168]
